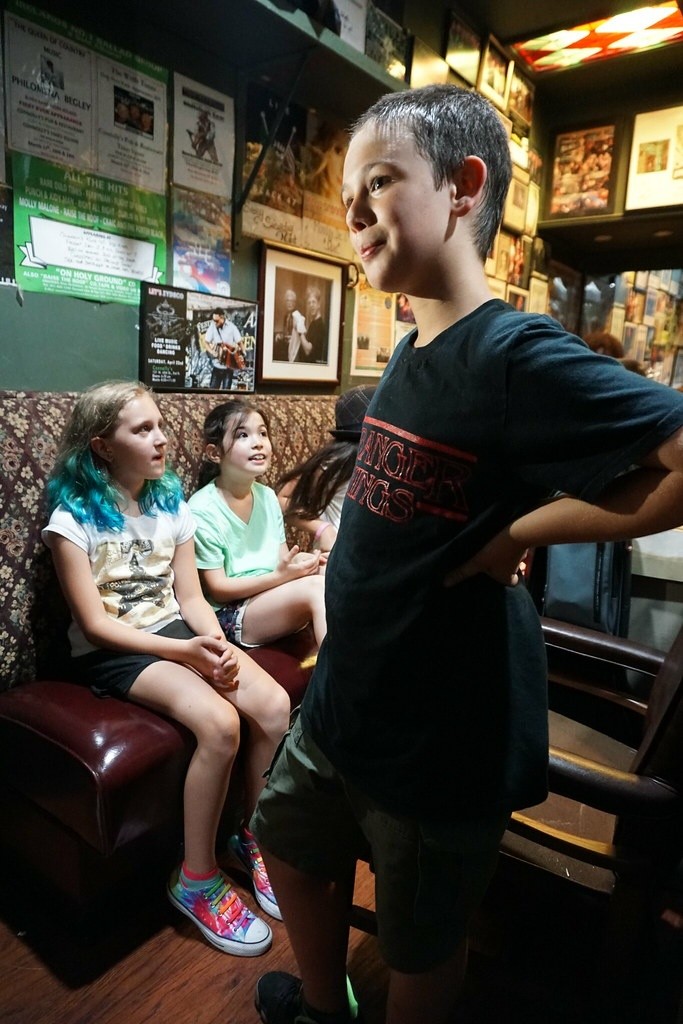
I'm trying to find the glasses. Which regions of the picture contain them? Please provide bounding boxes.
[214,318,222,322]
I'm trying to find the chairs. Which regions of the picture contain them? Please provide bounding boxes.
[342,612,683,1008]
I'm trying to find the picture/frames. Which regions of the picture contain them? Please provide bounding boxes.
[475,32,517,112]
[445,11,482,86]
[624,100,683,214]
[410,36,449,89]
[255,237,349,386]
[484,221,683,389]
[503,177,529,231]
[508,58,536,129]
[140,280,259,394]
[538,104,638,232]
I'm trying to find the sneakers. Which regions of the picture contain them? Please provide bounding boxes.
[224,828,285,921]
[165,868,273,956]
[256,970,352,1024]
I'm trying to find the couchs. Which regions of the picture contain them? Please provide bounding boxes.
[0,386,339,917]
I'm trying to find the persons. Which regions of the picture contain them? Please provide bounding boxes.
[253,79,682,1022]
[40,374,295,957]
[205,306,245,392]
[185,399,331,651]
[272,283,331,364]
[277,383,378,576]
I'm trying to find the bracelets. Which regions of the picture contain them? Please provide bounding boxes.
[315,521,333,542]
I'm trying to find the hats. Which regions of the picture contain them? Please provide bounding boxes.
[330,385,376,439]
